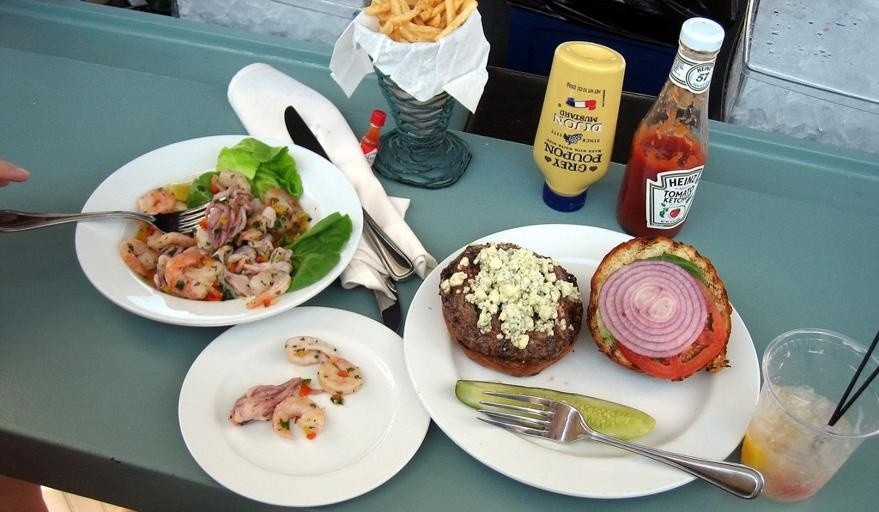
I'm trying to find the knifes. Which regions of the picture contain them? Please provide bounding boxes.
[283,106,417,283]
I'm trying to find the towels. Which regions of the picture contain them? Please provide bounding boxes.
[226,60,440,319]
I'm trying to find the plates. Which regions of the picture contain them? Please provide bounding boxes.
[175,302,433,509]
[399,220,762,501]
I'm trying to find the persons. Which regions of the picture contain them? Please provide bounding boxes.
[0,160,49,512]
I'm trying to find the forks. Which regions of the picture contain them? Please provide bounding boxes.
[0,194,228,237]
[476,389,765,502]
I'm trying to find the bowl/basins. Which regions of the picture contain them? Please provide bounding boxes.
[71,133,368,330]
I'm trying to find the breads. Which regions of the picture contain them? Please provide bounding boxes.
[588,236,731,373]
[438,239,585,376]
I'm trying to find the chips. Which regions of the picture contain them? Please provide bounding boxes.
[360,0,478,42]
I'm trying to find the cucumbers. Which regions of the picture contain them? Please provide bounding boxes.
[455,380,655,442]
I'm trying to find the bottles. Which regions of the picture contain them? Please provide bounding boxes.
[529,38,627,212]
[353,110,385,172]
[616,16,727,241]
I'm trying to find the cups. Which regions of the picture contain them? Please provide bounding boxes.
[737,328,879,505]
[352,2,483,190]
